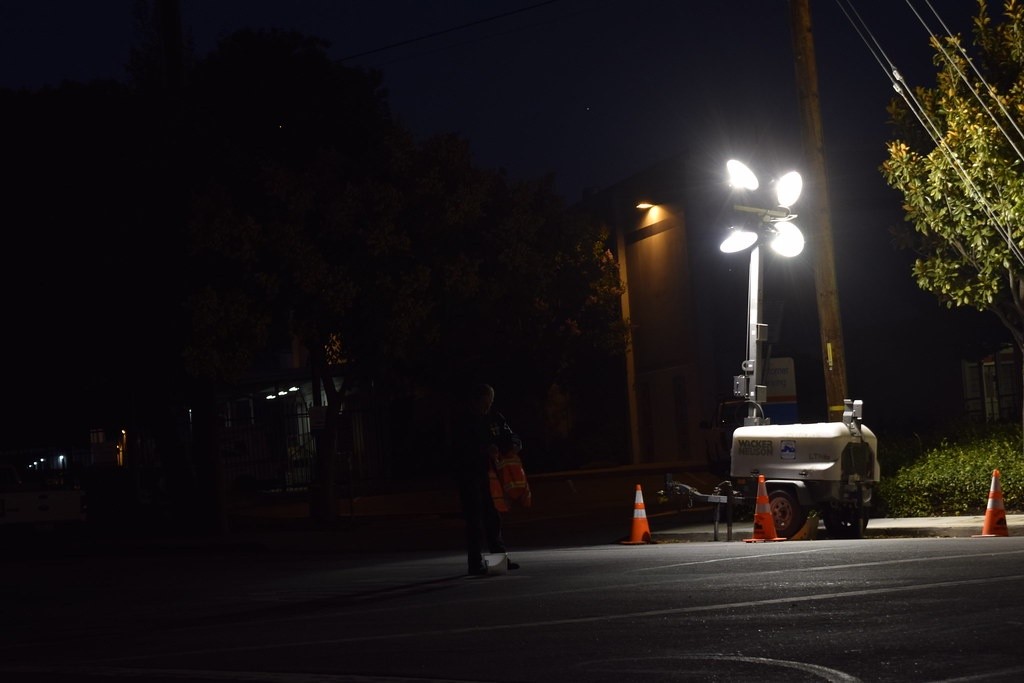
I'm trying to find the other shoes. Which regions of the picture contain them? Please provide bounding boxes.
[507,557,520,570]
[468,559,489,576]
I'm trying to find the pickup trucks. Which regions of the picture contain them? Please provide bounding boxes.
[0,461,89,559]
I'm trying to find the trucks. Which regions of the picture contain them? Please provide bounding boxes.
[137,421,281,497]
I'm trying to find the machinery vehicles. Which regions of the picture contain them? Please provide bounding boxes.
[658,397,881,543]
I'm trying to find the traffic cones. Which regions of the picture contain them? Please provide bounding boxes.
[742,474,788,542]
[620,484,663,545]
[971,469,1013,537]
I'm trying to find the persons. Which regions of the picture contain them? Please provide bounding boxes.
[451,384,524,581]
